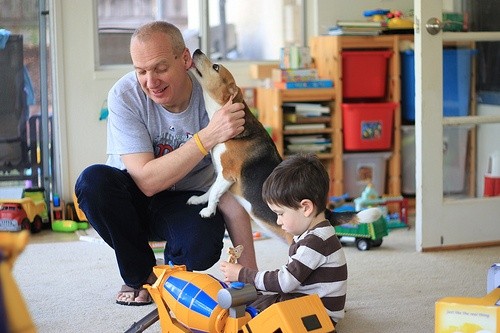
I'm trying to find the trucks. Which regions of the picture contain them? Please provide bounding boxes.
[324,207,389,252]
[0,198,44,234]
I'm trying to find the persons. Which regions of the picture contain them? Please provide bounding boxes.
[75,21,258,306]
[220,153,348,328]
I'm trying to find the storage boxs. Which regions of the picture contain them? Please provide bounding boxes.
[342,50,392,97]
[434,287,500,333]
[340,102,397,151]
[344,152,392,198]
[401,49,477,121]
[402,125,475,194]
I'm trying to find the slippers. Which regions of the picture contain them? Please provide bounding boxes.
[115,272,158,306]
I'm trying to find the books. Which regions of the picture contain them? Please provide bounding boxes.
[282,102,333,155]
[328,20,383,36]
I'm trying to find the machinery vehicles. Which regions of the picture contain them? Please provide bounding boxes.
[141,260,336,333]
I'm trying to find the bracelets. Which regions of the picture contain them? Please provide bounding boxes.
[195,134,208,156]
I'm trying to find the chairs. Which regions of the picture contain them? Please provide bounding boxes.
[0,32,43,190]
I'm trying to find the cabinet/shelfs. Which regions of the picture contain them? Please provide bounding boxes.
[312,36,479,215]
[255,87,340,202]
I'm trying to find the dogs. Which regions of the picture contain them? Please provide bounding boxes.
[187,49,294,247]
